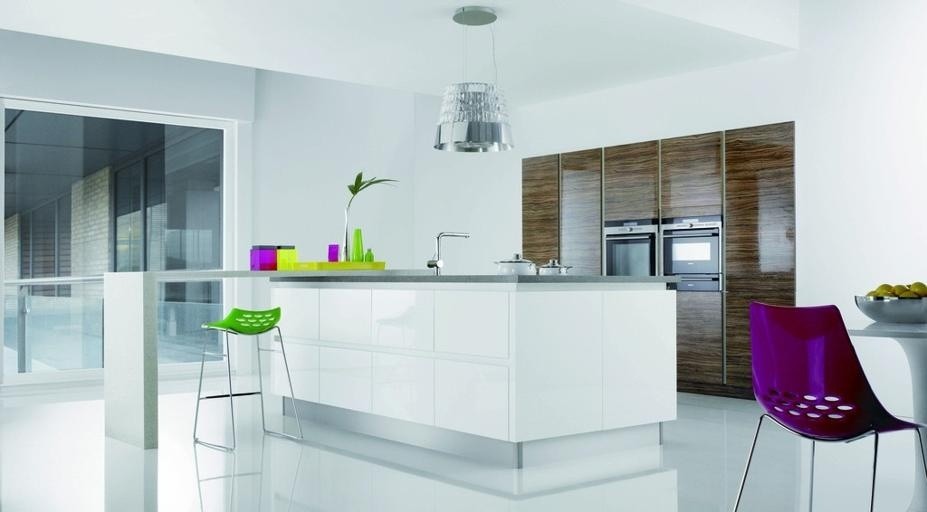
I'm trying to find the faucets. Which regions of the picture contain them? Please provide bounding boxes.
[426,232,470,276]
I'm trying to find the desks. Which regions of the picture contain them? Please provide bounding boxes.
[841,313,927,512]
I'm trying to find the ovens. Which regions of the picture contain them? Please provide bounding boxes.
[603,218,661,277]
[661,214,723,293]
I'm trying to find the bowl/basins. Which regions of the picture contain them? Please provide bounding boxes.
[854,295,927,325]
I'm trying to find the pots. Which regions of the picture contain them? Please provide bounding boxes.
[536,258,574,276]
[492,251,538,277]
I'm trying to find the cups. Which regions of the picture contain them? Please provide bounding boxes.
[327,243,340,262]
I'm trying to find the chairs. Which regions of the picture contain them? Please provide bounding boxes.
[733,301,927,512]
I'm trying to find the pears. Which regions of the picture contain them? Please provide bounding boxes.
[867,282,927,297]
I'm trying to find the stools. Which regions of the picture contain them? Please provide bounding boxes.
[193,307,305,450]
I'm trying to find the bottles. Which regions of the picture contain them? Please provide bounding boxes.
[351,228,365,263]
[364,249,375,263]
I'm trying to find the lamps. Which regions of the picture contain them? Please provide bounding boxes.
[434,5,516,154]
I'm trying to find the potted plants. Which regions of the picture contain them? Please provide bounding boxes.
[341,172,399,262]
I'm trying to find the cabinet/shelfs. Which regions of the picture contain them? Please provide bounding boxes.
[661,131,724,397]
[603,138,660,277]
[522,152,559,267]
[558,146,603,276]
[724,119,794,401]
